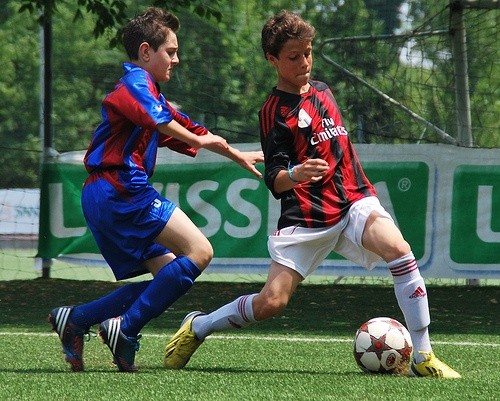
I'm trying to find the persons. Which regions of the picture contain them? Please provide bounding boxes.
[164,8,462,379]
[47,6,265,373]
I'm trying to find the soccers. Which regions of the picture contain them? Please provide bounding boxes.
[352,317,413,374]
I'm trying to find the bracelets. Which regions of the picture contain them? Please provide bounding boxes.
[287,159,302,184]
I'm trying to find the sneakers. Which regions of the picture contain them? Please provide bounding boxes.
[98,316,142,372]
[409,351,461,379]
[48,306,97,372]
[165,311,207,369]
